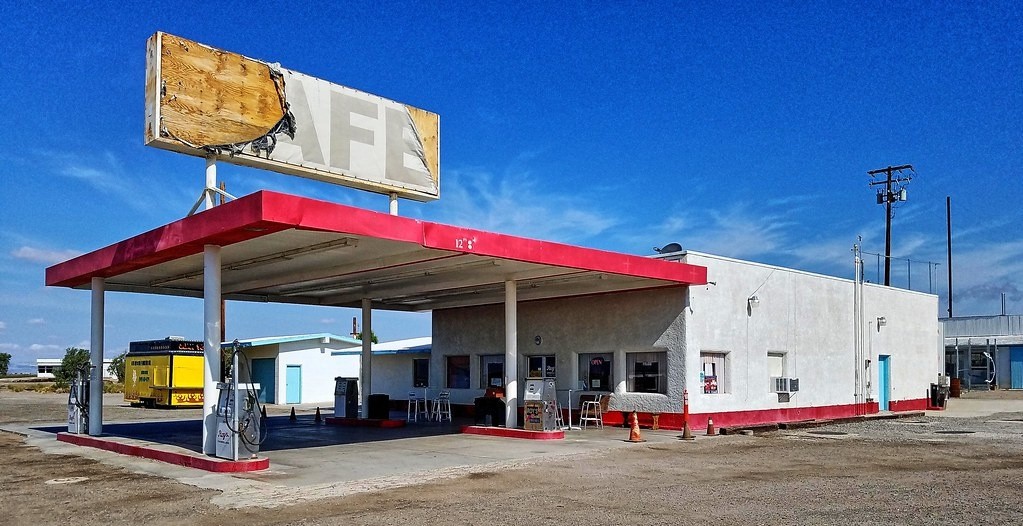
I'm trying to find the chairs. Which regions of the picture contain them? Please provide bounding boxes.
[429,391,451,422]
[407,392,429,422]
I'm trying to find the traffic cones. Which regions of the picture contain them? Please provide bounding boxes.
[623,411,647,442]
[261,405,269,419]
[288,407,298,421]
[314,407,322,421]
[679,421,696,439]
[703,416,719,435]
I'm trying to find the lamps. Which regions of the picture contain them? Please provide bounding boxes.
[748,296,759,309]
[877,316,886,326]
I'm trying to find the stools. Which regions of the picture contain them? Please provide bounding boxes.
[579,394,604,430]
[556,402,564,428]
